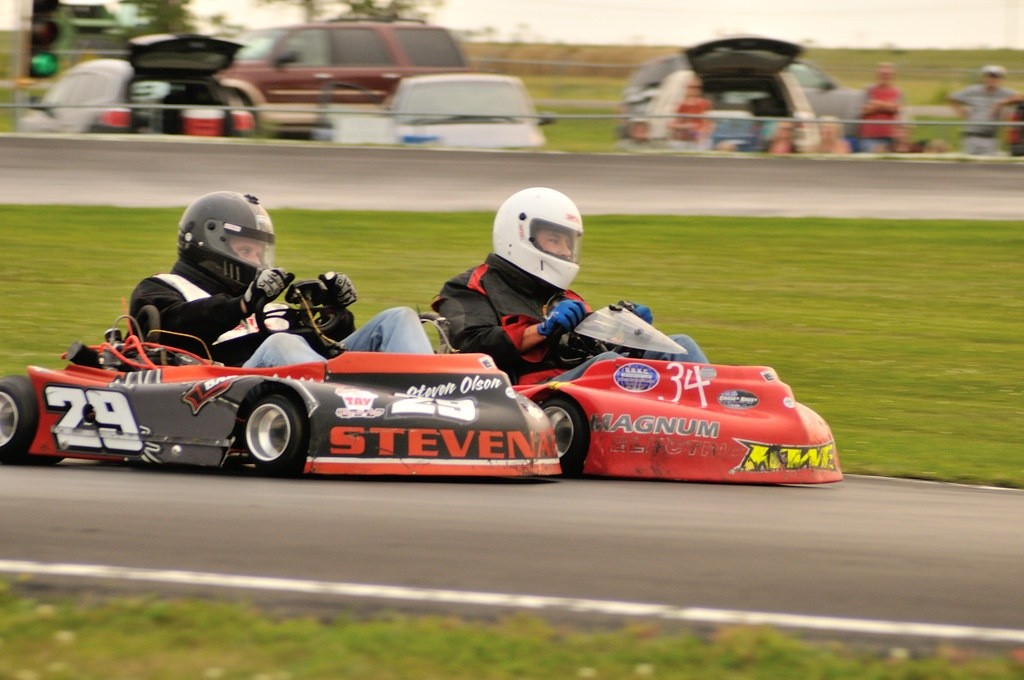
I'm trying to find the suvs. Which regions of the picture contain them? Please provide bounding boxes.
[210,18,476,125]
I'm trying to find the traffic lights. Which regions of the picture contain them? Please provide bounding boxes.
[30,0,60,76]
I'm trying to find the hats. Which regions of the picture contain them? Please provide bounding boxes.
[983,66,1005,73]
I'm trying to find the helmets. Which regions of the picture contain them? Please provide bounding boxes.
[176,190,274,297]
[485,188,583,297]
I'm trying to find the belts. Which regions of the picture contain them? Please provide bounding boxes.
[966,132,994,138]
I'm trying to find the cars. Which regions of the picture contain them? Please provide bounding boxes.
[389,74,556,150]
[614,35,870,154]
[119,33,247,138]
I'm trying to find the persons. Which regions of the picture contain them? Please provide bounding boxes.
[130,191,434,368]
[432,187,710,382]
[614,64,1024,154]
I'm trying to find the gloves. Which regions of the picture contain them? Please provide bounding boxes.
[538,300,586,336]
[618,301,653,326]
[320,271,357,307]
[242,268,295,317]
